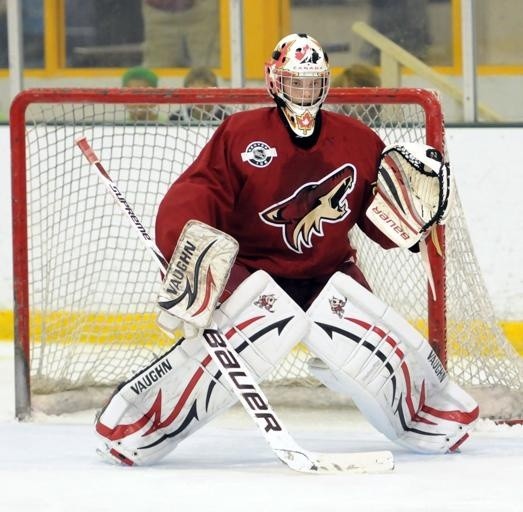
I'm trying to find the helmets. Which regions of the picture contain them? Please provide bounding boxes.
[265,32,331,111]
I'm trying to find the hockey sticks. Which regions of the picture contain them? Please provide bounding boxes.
[75,136,394,475]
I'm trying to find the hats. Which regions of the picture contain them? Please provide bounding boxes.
[120,63,157,88]
[182,64,219,88]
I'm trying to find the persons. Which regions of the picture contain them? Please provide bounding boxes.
[120,66,165,122]
[95,31,481,468]
[140,1,220,68]
[168,65,230,121]
[332,64,382,123]
